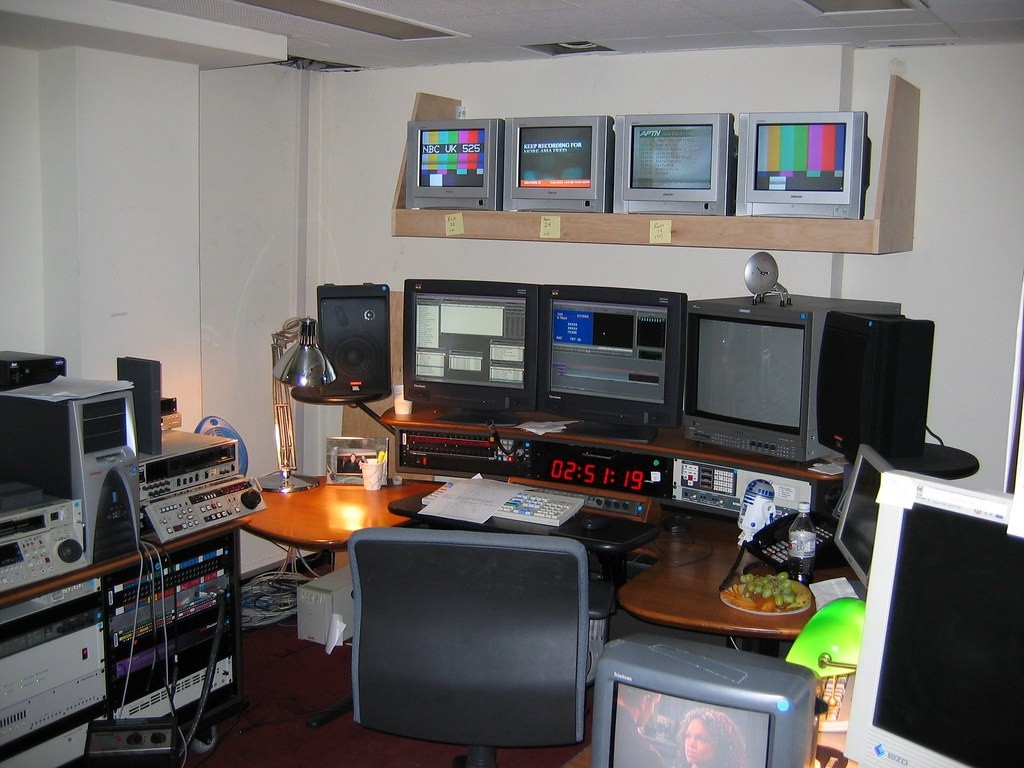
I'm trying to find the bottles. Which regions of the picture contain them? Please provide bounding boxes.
[789,502,816,584]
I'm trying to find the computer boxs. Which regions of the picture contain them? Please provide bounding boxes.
[0,381,141,565]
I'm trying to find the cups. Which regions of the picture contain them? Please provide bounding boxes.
[394,385,412,414]
[361,459,385,490]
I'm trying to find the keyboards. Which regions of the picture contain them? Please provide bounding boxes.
[814,670,856,732]
[421,478,585,527]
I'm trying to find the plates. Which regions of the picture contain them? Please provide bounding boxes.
[720,580,811,616]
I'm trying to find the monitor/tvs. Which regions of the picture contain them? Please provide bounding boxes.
[834,444,897,602]
[402,279,539,427]
[536,285,690,443]
[406,112,872,221]
[591,633,819,768]
[843,471,1024,768]
[684,293,901,462]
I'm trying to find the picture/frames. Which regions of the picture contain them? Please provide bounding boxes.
[327,437,388,488]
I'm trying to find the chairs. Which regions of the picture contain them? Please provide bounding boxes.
[349,525,617,768]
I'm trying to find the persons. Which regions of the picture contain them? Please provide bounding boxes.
[613,683,667,768]
[673,708,747,768]
[344,453,368,472]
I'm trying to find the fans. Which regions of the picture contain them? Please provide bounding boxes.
[193,415,248,476]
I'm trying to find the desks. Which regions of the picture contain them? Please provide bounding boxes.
[0,516,252,768]
[237,473,859,730]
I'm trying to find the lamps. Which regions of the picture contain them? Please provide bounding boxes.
[255,317,337,493]
[785,597,866,678]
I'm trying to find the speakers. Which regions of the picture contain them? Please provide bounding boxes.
[817,310,935,458]
[317,282,391,396]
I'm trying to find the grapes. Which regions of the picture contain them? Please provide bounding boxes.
[740,571,796,606]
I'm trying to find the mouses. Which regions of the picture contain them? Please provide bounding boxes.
[582,513,617,529]
